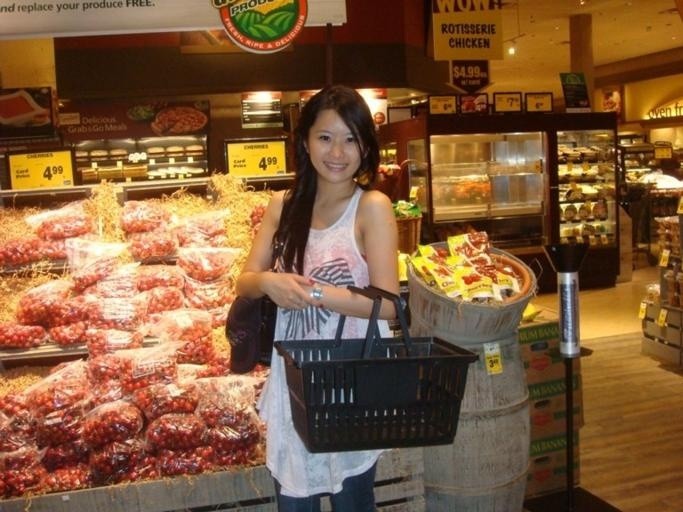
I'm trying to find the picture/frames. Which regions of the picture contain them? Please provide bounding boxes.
[427,91,554,113]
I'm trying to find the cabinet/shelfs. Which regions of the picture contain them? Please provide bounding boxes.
[0,110,683,512]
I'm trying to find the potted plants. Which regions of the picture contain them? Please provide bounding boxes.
[427,91,554,113]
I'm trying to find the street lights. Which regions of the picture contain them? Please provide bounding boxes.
[226,297,277,373]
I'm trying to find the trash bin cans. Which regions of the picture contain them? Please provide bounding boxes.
[311,282,323,301]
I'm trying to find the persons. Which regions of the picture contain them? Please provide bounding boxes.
[236,85,406,512]
